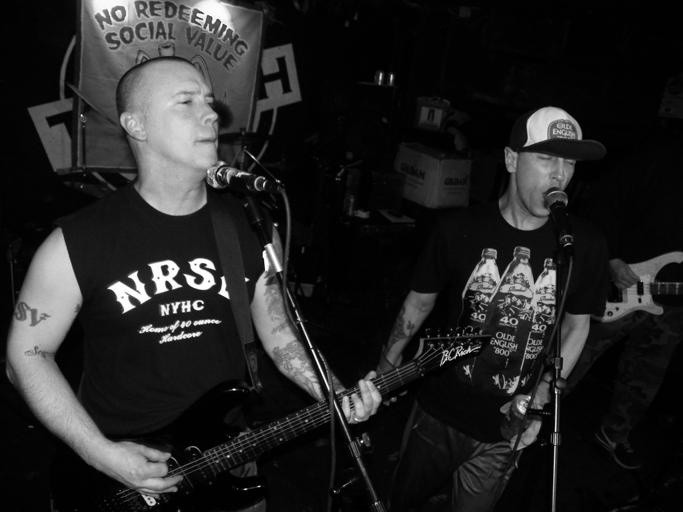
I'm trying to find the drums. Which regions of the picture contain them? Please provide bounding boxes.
[240,192,281,226]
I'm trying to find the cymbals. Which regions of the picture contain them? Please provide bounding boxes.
[219,133,272,145]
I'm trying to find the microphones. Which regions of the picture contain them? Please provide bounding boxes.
[543,186,575,252]
[205,160,267,194]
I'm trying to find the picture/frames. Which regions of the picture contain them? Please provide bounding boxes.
[412,95,450,134]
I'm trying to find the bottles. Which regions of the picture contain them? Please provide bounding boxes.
[453,242,563,399]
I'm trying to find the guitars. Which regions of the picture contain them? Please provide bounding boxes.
[49,326,492,512]
[588,250,682,323]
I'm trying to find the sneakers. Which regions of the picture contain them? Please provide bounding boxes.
[596,426,641,470]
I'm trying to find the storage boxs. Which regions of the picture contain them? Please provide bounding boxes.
[403,141,472,211]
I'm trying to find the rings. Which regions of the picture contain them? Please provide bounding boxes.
[354,416,363,423]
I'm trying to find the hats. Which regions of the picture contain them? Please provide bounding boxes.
[506,106,606,162]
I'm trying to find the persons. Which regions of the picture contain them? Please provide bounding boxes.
[376,104,610,511]
[6,57,384,512]
[561,122,682,470]
